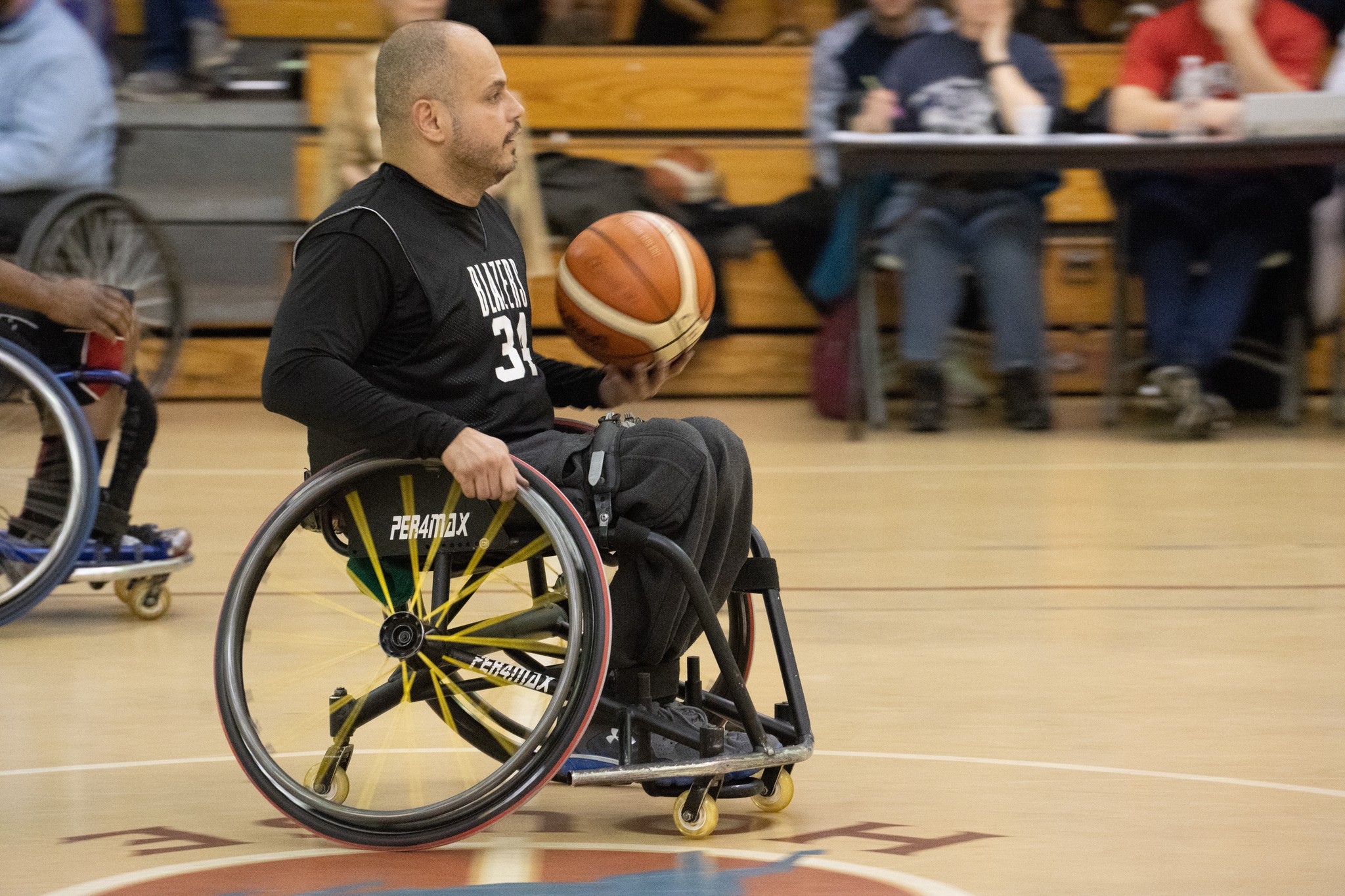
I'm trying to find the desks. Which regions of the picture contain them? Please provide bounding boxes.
[830,129,1345,441]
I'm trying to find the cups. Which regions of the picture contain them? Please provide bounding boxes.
[1015,103,1051,137]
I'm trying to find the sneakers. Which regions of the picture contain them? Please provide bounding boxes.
[558,699,783,787]
[6,509,191,567]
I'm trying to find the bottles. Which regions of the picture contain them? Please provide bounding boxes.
[1172,54,1204,139]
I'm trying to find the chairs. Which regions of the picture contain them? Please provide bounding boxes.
[1109,89,1301,427]
[857,73,1042,431]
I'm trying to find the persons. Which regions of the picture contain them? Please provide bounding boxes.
[251,20,754,769]
[1,0,230,580]
[436,0,1345,444]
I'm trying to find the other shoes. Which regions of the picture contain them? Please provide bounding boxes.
[1120,362,1235,438]
[1002,369,1052,428]
[906,364,946,433]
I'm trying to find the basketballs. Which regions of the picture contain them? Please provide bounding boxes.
[555,210,715,372]
[646,146,727,203]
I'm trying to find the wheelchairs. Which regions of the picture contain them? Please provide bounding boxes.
[0,286,193,631]
[211,420,818,856]
[0,123,189,400]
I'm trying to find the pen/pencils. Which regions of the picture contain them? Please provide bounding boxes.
[1132,127,1174,139]
[862,76,902,116]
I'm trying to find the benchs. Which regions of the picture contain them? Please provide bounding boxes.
[104,0,1345,93]
